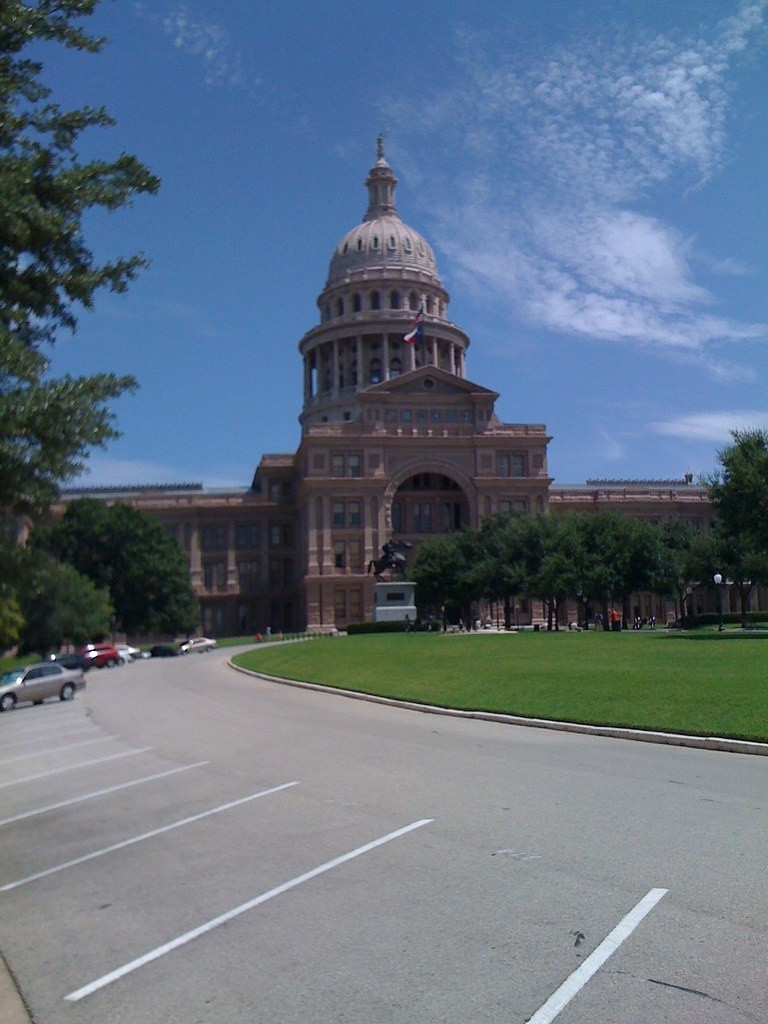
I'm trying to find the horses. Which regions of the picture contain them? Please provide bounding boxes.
[367,540,414,581]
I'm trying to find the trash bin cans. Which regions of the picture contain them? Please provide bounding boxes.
[330,628,339,637]
[613,619,621,631]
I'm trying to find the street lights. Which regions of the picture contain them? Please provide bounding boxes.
[713,572,725,631]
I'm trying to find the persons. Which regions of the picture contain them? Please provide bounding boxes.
[255,625,272,643]
[401,612,656,633]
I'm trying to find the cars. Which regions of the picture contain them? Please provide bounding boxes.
[148,643,181,657]
[115,645,142,663]
[3,653,92,688]
[180,637,217,653]
[0,662,87,710]
[78,643,121,669]
